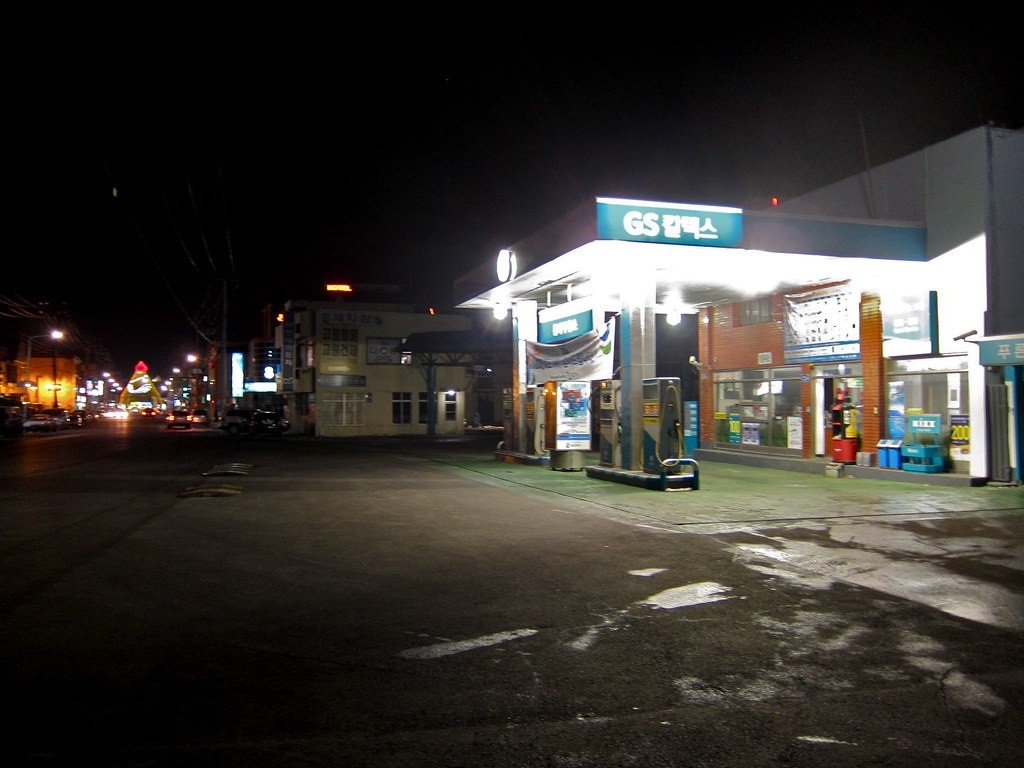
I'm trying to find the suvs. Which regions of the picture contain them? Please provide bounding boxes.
[221,410,291,438]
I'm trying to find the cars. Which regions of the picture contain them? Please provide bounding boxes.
[0,400,100,433]
[166,408,209,430]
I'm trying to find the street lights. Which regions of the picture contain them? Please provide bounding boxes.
[24,330,64,433]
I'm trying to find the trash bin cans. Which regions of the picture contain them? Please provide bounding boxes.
[876,438,904,470]
